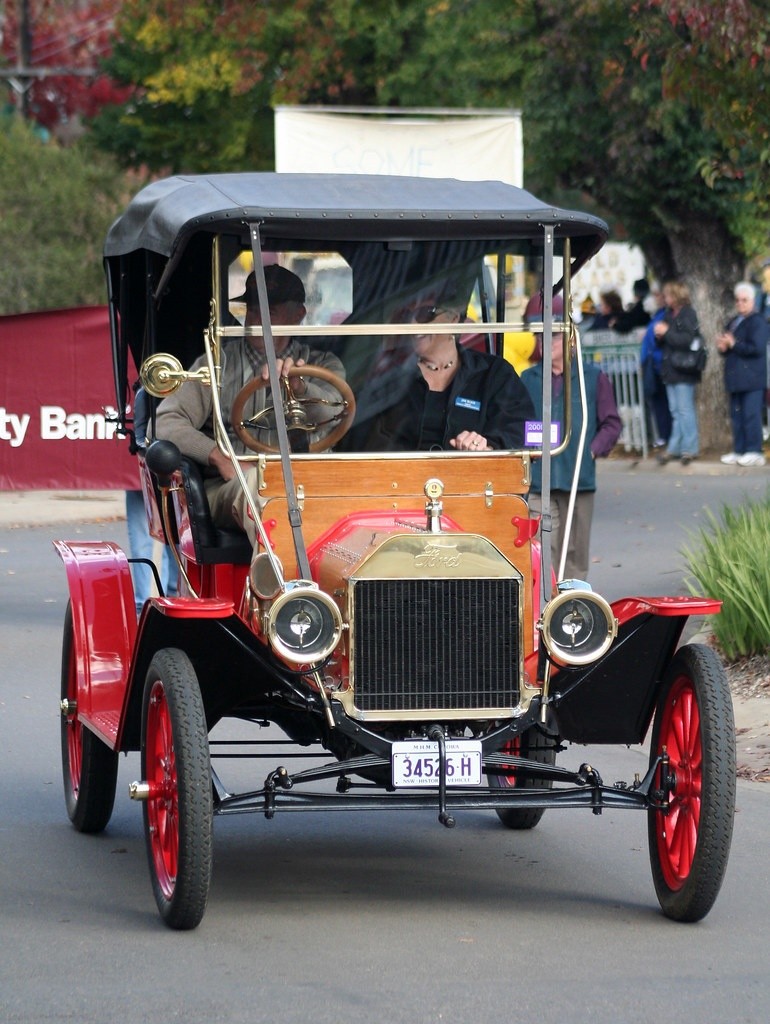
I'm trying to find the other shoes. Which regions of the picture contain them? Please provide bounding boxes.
[720,450,743,465]
[680,453,695,466]
[651,436,666,447]
[735,451,767,467]
[657,453,676,464]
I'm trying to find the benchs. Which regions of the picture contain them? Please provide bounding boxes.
[134,377,252,567]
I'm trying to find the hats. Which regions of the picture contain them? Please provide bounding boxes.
[227,263,305,303]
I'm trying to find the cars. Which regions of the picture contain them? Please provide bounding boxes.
[49,173,737,930]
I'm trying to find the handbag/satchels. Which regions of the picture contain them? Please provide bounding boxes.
[671,335,706,372]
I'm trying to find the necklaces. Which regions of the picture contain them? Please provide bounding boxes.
[422,360,454,371]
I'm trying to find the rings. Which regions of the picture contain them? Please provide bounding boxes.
[472,441,479,446]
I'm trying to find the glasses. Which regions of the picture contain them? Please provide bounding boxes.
[735,298,750,304]
[396,304,446,323]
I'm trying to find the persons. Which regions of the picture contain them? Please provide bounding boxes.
[515,315,625,581]
[716,282,770,467]
[639,279,699,464]
[125,490,179,614]
[593,279,652,407]
[146,263,346,548]
[335,287,536,453]
